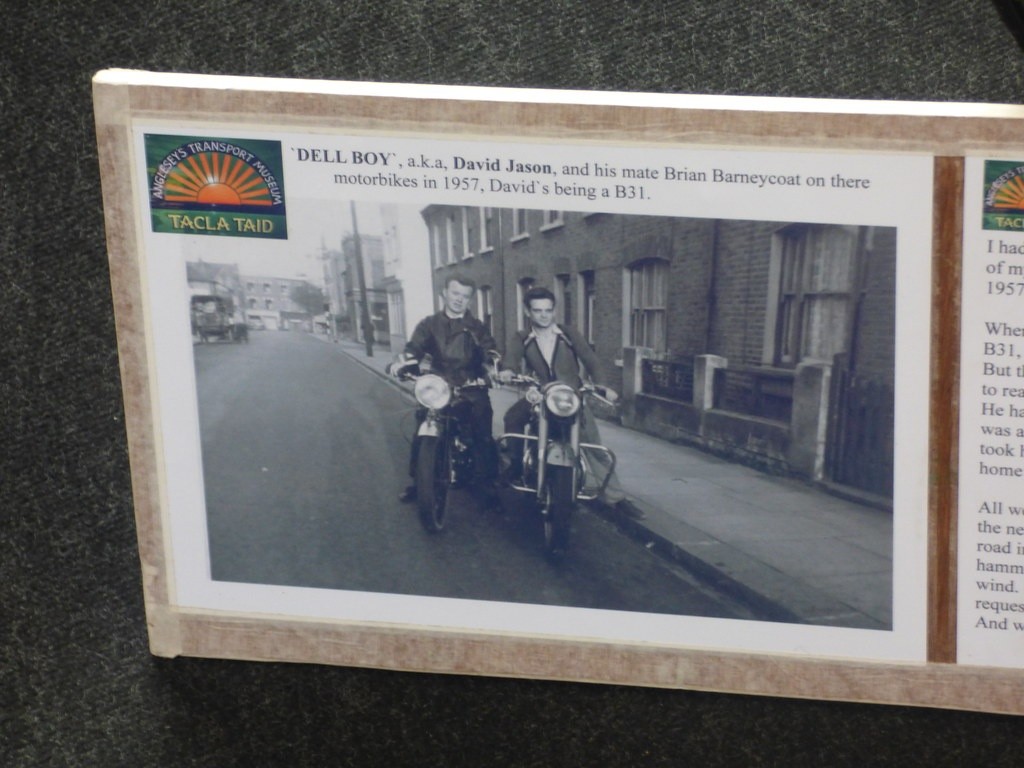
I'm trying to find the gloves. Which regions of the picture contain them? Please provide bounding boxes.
[453,369,492,397]
[388,352,420,381]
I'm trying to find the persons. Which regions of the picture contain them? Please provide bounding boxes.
[498,287,647,519]
[391,273,506,512]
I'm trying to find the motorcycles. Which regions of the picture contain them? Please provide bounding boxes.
[384,348,621,562]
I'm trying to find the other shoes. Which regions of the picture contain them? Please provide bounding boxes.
[398,485,418,502]
[616,498,647,519]
[500,464,523,488]
[471,486,504,513]
